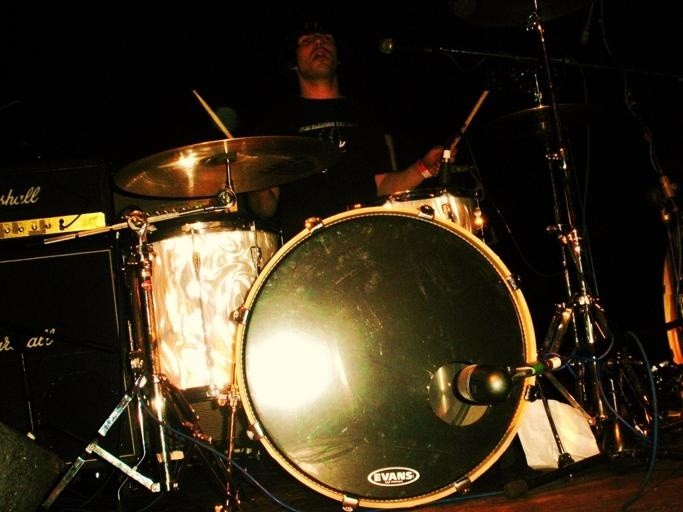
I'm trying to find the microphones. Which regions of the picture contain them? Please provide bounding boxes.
[452,363,514,407]
[379,37,432,55]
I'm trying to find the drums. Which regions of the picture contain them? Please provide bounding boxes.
[143,213,280,404]
[392,187,475,237]
[230,207,538,509]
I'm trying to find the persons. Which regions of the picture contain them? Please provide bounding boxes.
[245,21,458,244]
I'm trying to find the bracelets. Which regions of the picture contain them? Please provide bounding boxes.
[416,158,432,179]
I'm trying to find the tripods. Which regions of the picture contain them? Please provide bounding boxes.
[41,249,246,512]
[504,16,683,499]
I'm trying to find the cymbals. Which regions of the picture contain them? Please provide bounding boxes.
[501,103,589,123]
[116,135,338,197]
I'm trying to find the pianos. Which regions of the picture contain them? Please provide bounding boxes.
[1,211,107,239]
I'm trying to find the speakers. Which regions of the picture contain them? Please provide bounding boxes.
[0,231,142,468]
